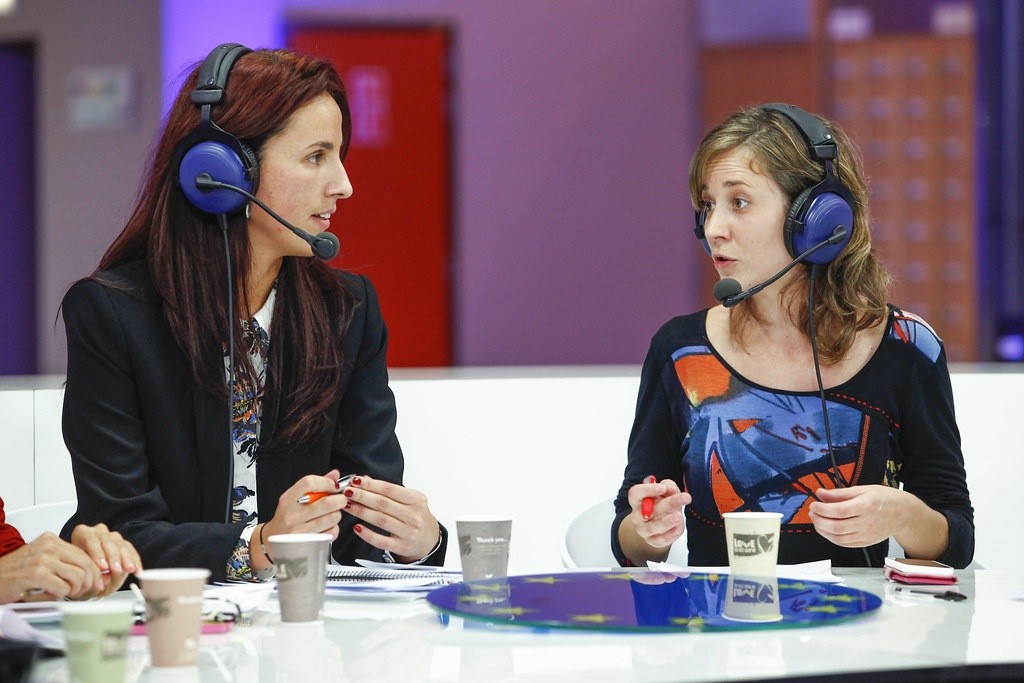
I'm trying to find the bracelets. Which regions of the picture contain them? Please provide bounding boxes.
[258,522,273,568]
[382,528,443,564]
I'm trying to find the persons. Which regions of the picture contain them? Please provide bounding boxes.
[608,104,976,572]
[51,43,447,591]
[1,497,143,606]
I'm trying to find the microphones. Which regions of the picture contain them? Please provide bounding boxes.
[195,172,339,260]
[714,225,844,306]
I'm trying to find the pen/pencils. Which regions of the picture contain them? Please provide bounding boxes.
[20,569,112,597]
[895,587,968,603]
[297,473,358,504]
[641,475,656,520]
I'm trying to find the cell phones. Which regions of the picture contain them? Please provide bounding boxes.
[27,568,132,603]
[885,558,954,575]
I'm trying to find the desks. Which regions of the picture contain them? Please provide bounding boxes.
[1,563,1024,681]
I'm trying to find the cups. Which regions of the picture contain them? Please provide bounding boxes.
[137,566,210,671]
[457,518,512,585]
[268,532,332,626]
[60,598,132,683]
[723,511,783,578]
[721,568,784,625]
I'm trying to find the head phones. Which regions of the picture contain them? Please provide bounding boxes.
[695,103,855,264]
[171,42,260,217]
